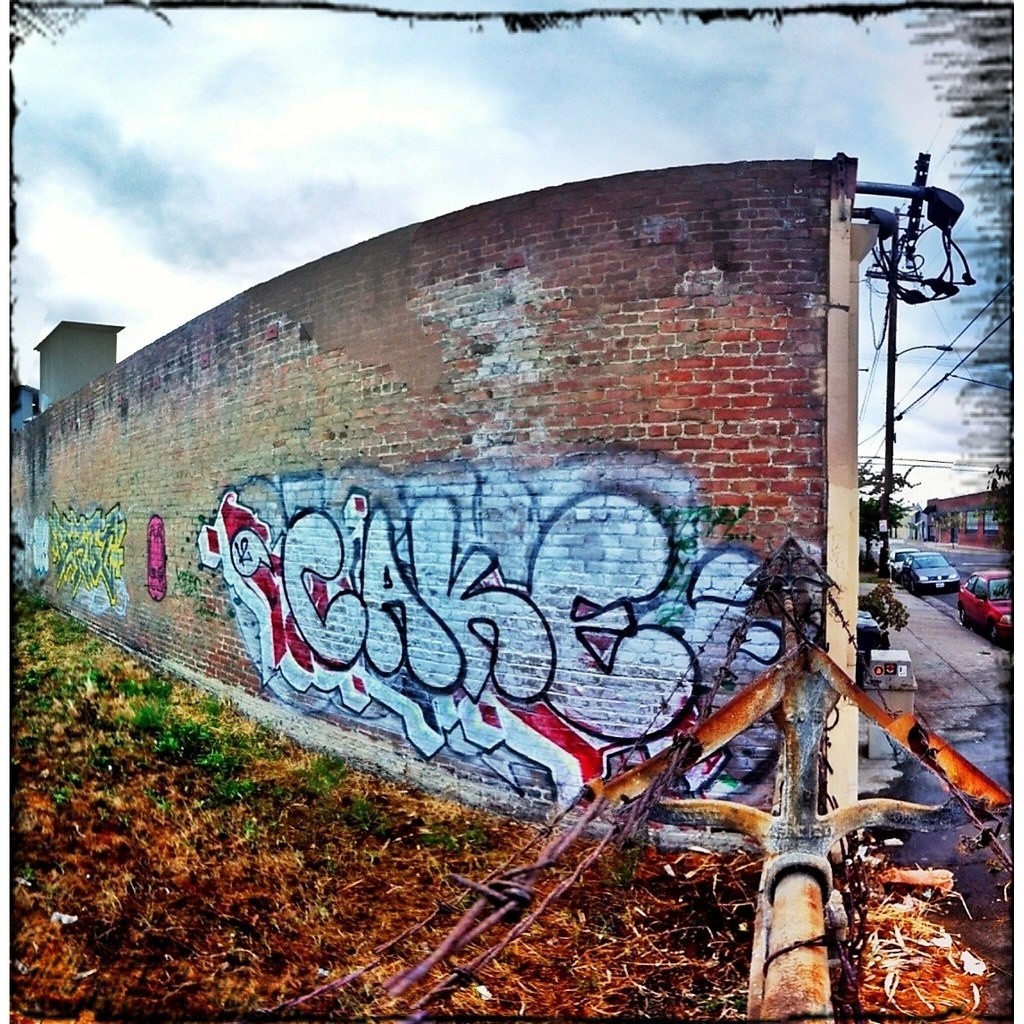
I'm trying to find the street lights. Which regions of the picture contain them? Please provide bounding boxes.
[877,345,953,582]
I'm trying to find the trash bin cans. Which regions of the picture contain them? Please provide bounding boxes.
[862,649,918,759]
[856,610,891,693]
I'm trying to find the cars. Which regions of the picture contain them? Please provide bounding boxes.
[956,570,1014,646]
[889,548,921,579]
[899,551,961,595]
